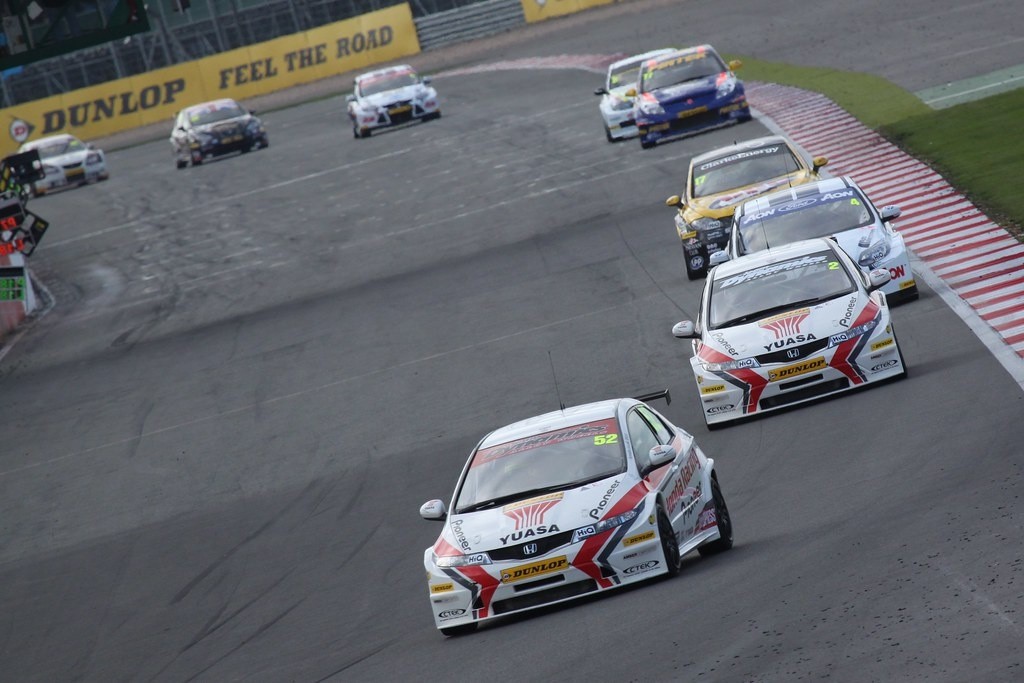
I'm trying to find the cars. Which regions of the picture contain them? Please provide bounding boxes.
[625,44,751,149]
[418,389,735,637]
[168,97,269,168]
[709,174,919,301]
[347,65,441,138]
[19,132,108,198]
[672,236,907,432]
[666,134,829,280]
[594,47,678,143]
